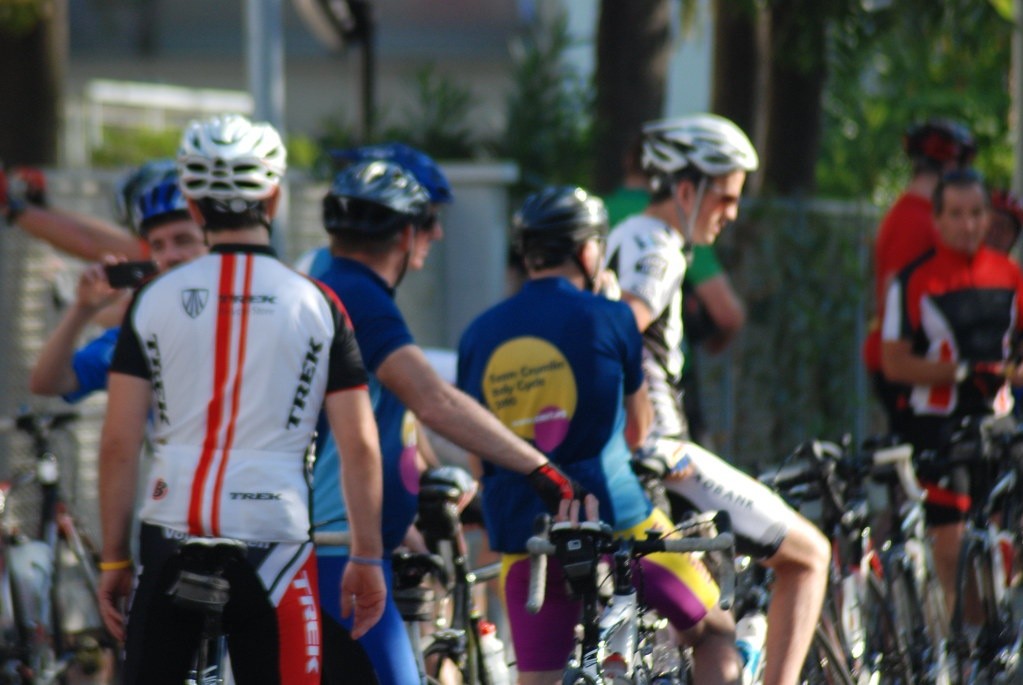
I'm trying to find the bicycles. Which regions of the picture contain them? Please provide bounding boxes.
[0,418,1023,684]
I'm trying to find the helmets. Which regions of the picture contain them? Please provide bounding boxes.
[642,115,757,178]
[178,118,285,203]
[990,189,1023,233]
[351,143,453,204]
[126,167,196,231]
[906,120,976,169]
[323,161,430,237]
[509,188,609,252]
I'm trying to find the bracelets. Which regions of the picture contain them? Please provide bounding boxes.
[349,553,384,566]
[102,560,133,571]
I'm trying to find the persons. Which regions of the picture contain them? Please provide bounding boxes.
[0,107,1023,685]
[94,121,387,685]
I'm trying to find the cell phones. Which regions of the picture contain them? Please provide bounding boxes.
[104,260,161,290]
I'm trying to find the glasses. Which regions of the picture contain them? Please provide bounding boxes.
[708,186,738,206]
[421,213,439,232]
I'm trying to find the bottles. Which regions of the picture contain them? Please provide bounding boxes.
[476,619,511,685]
[735,606,769,685]
